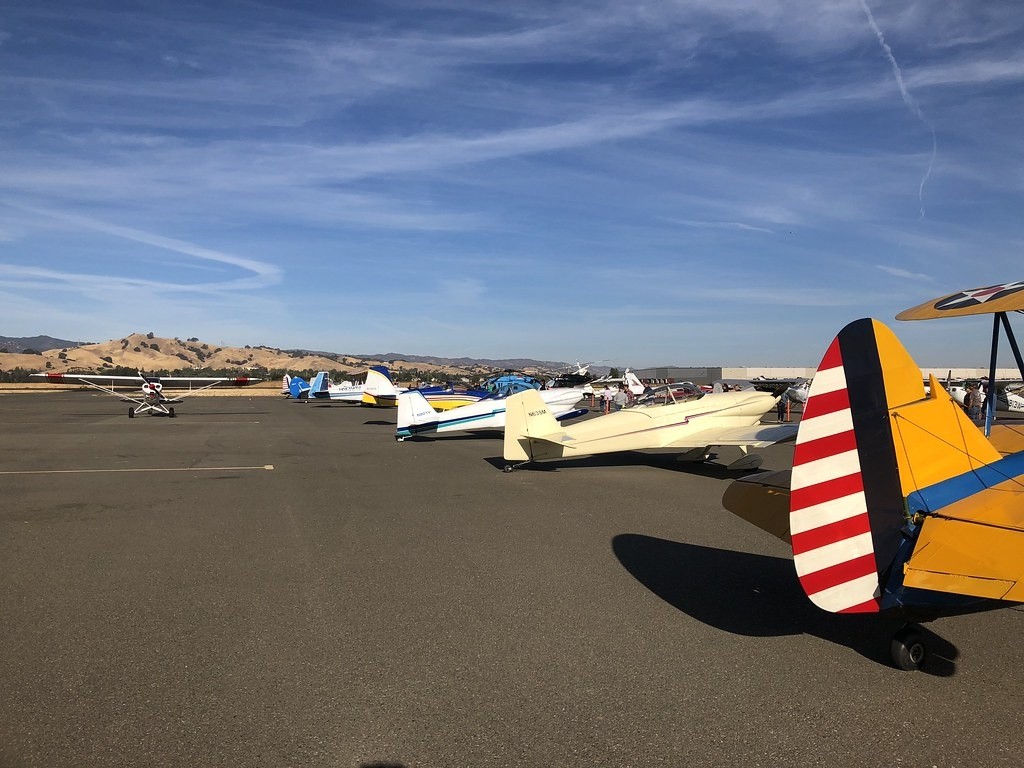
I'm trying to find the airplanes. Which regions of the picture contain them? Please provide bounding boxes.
[709,375,813,404]
[360,365,482,413]
[789,316,1024,669]
[393,388,588,444]
[791,373,1023,415]
[570,359,593,376]
[307,371,454,407]
[502,383,796,481]
[27,371,262,418]
[281,374,362,400]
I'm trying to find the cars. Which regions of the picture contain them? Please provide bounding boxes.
[635,381,704,406]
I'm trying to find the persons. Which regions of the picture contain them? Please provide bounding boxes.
[721,383,730,393]
[623,384,635,405]
[603,385,612,413]
[776,392,788,424]
[642,381,653,395]
[539,379,546,391]
[598,395,604,414]
[507,387,513,396]
[963,387,971,416]
[485,382,493,393]
[613,386,628,412]
[968,384,981,420]
[513,386,518,394]
[734,384,741,392]
